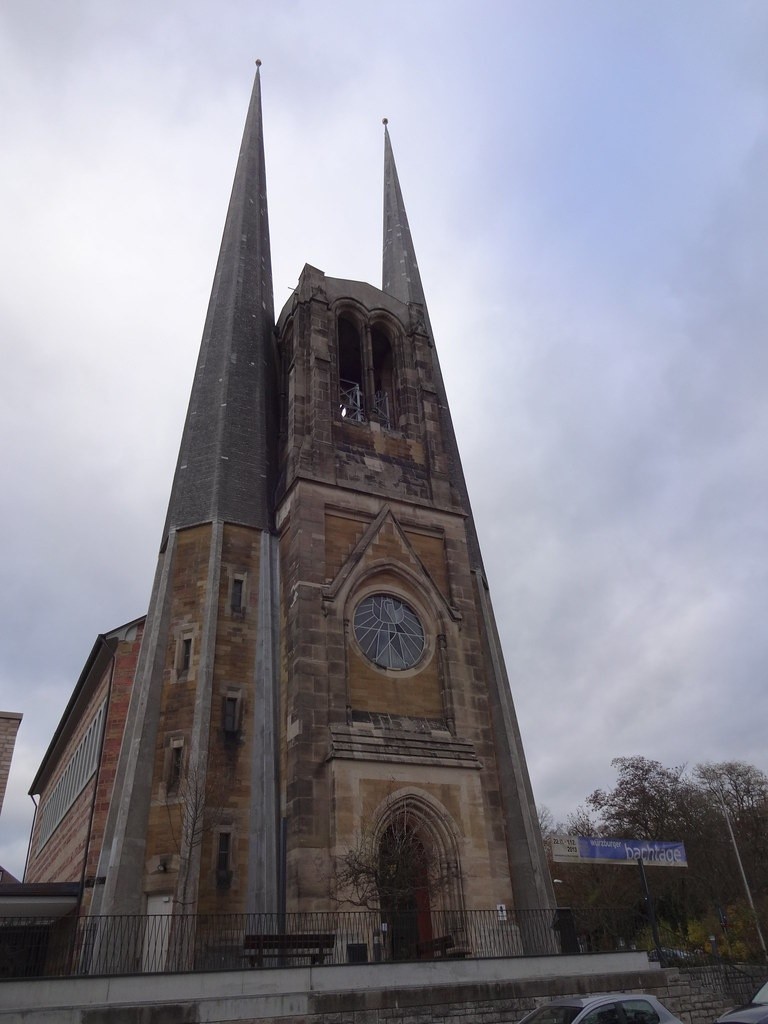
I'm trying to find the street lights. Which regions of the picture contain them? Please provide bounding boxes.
[693,767,768,963]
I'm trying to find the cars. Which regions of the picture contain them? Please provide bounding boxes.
[650,949,692,964]
[518,993,684,1024]
[713,980,768,1024]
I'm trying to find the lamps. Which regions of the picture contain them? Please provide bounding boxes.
[157,861,167,872]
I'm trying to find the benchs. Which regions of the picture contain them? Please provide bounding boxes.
[416,935,472,959]
[241,935,335,967]
